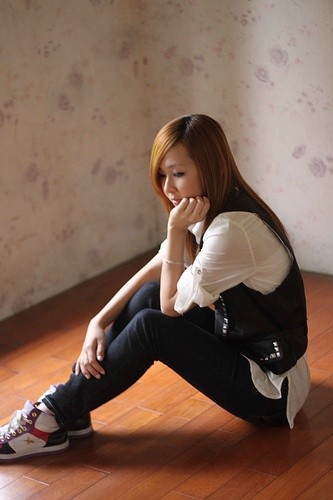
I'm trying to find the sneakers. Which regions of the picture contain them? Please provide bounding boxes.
[36,383,93,439]
[0,400,69,459]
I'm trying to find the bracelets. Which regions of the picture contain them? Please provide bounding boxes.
[162,258,184,265]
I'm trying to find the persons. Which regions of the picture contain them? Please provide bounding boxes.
[0,114,311,462]
